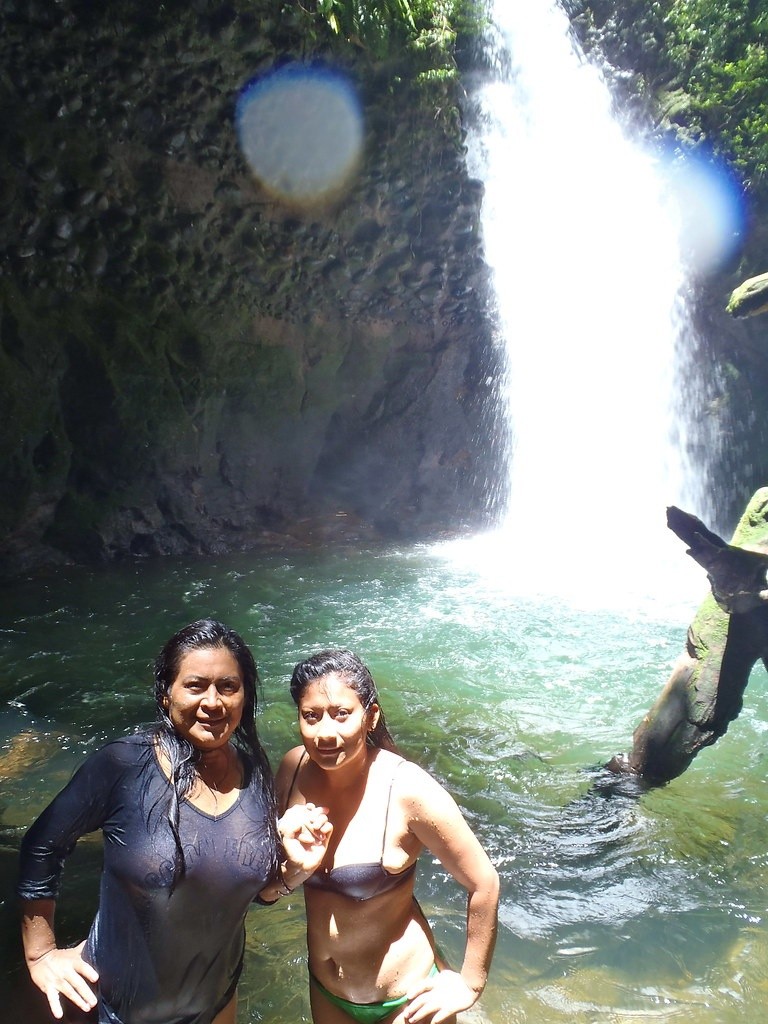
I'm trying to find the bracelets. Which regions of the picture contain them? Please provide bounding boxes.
[276,863,296,897]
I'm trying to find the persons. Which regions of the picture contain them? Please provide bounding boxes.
[16,620,332,1024]
[271,650,502,1024]
